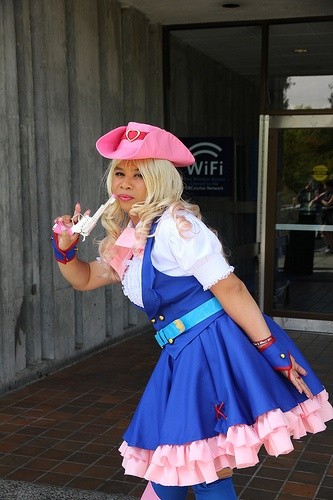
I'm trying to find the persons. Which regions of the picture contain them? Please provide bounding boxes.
[51,120,332,500]
[276,172,332,259]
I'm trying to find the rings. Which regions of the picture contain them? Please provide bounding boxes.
[295,377,301,381]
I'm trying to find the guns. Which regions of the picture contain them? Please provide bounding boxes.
[53,194,118,241]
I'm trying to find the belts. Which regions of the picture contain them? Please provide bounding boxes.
[155,296,224,346]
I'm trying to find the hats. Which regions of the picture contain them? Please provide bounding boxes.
[95,122,195,167]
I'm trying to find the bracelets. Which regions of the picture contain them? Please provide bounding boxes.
[252,337,273,346]
